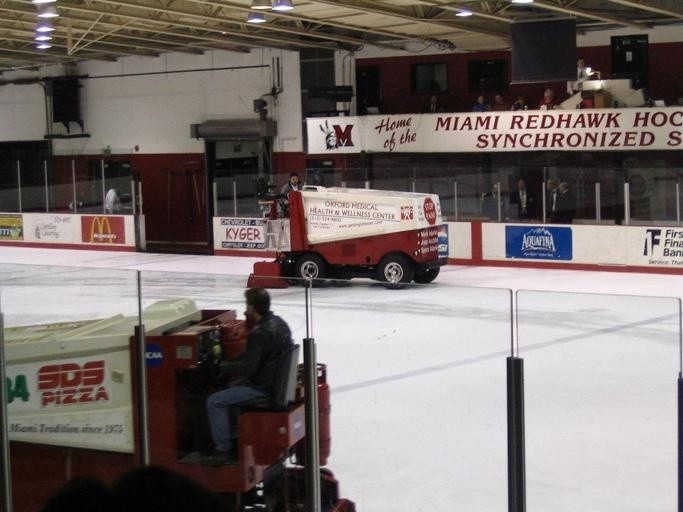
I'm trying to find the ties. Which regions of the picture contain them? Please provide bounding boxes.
[432,104,434,112]
[520,189,525,208]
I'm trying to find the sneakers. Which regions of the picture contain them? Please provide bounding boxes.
[209,447,235,465]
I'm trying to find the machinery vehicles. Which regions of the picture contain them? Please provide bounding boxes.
[243,183,450,290]
[1,297,333,512]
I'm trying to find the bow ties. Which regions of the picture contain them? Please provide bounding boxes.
[552,191,555,193]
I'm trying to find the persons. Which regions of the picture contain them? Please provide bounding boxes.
[630,175,652,221]
[425,95,443,113]
[278,172,303,217]
[546,176,576,223]
[314,172,324,186]
[577,56,585,80]
[201,288,295,464]
[481,178,536,219]
[473,92,529,112]
[536,87,563,110]
[106,184,120,214]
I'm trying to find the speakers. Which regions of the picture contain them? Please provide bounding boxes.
[52,79,79,122]
